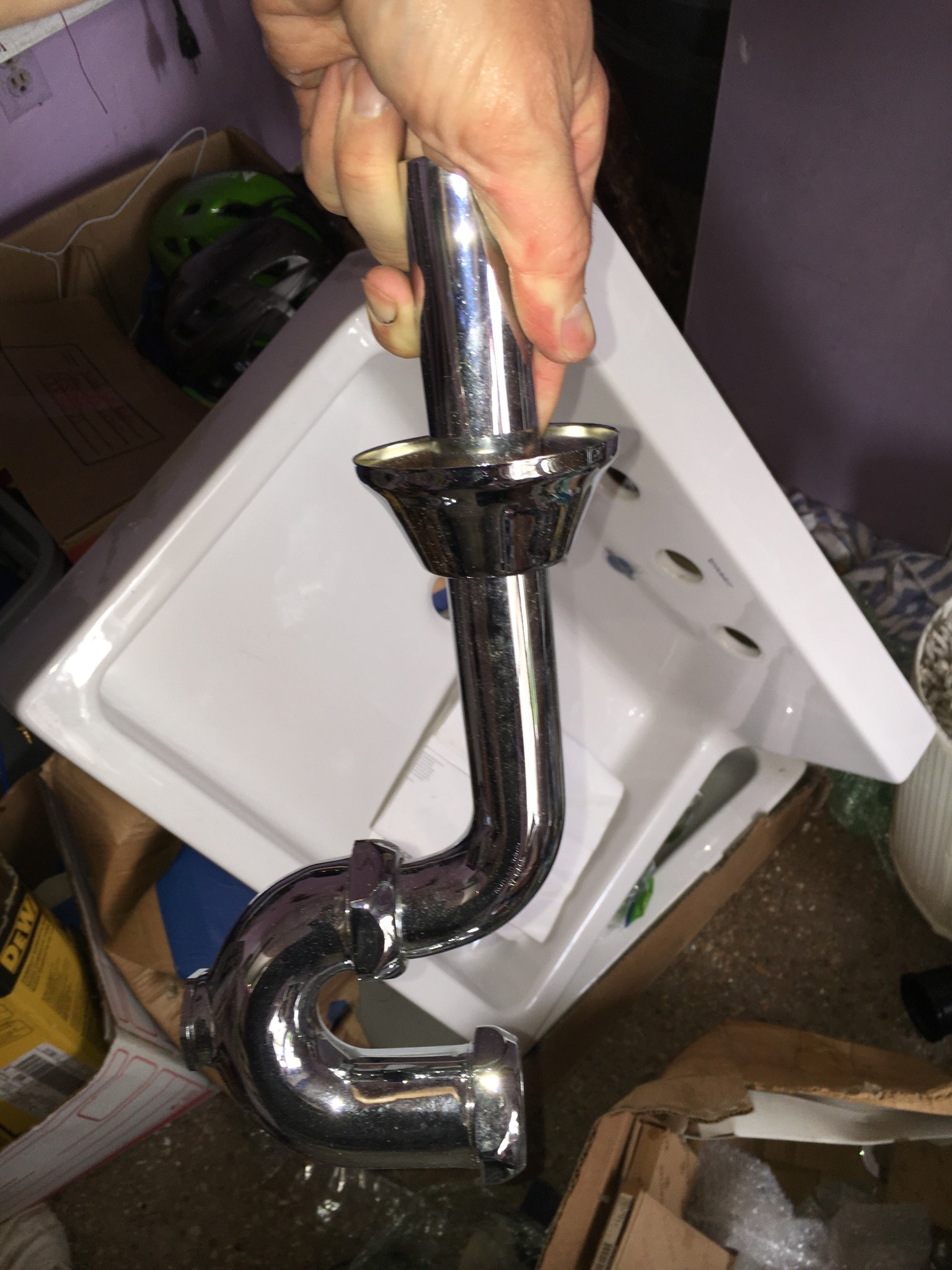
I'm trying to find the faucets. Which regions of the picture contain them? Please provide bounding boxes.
[154,155,619,1194]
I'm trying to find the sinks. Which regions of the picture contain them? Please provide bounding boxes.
[13,203,940,1065]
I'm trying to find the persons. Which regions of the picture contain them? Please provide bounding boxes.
[0,0,609,438]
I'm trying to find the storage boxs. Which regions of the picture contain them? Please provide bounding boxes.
[538,1026,952,1270]
[0,770,223,1217]
[0,129,367,558]
[525,763,839,1092]
